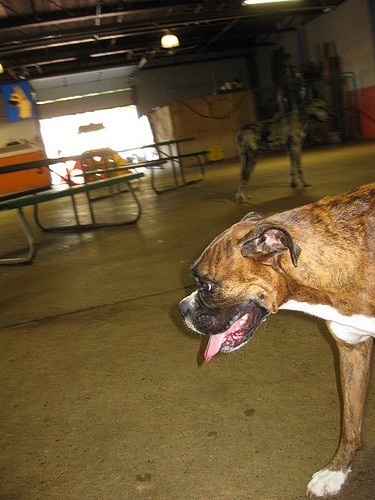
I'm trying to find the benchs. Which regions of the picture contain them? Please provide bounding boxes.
[0,172,145,268]
[75,150,211,202]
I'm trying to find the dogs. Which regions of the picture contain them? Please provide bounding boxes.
[178,183,375,496]
[235,98,333,202]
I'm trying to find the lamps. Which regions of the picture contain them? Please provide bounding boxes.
[160,29,179,49]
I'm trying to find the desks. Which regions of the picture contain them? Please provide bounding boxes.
[82,137,196,195]
[0,152,114,224]
